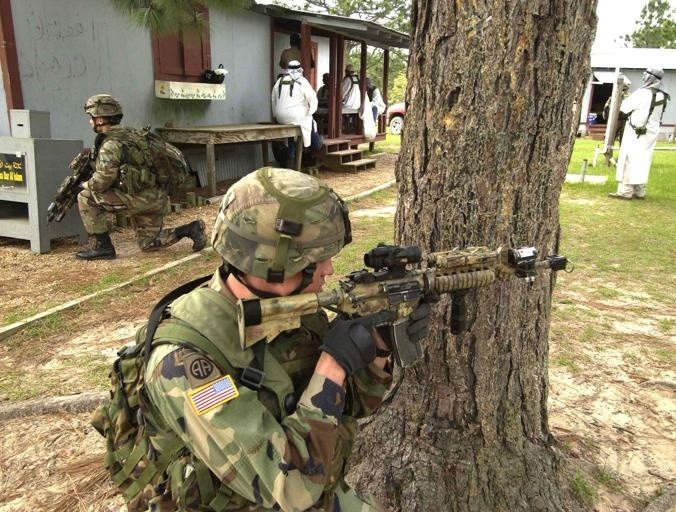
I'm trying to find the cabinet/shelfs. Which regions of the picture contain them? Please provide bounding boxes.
[0,136,89,255]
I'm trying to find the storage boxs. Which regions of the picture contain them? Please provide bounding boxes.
[7,107,50,139]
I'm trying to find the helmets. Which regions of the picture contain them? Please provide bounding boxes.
[210,166,352,283]
[83,94,122,118]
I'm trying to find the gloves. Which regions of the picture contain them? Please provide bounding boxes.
[376,299,430,356]
[318,310,398,377]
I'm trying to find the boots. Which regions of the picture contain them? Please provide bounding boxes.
[174,220,207,252]
[75,230,116,260]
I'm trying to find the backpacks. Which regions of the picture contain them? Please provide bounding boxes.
[100,126,188,196]
[89,311,326,511]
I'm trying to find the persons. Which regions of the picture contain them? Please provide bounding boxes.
[600,79,633,168]
[268,59,387,166]
[608,63,668,201]
[51,92,209,262]
[86,166,579,510]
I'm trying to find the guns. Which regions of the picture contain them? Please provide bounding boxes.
[235,244,569,368]
[44,148,102,221]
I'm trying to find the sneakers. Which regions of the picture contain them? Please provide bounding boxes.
[609,192,632,199]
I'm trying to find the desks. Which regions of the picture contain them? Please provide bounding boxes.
[145,122,305,195]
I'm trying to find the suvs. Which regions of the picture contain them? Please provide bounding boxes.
[386,103,406,137]
